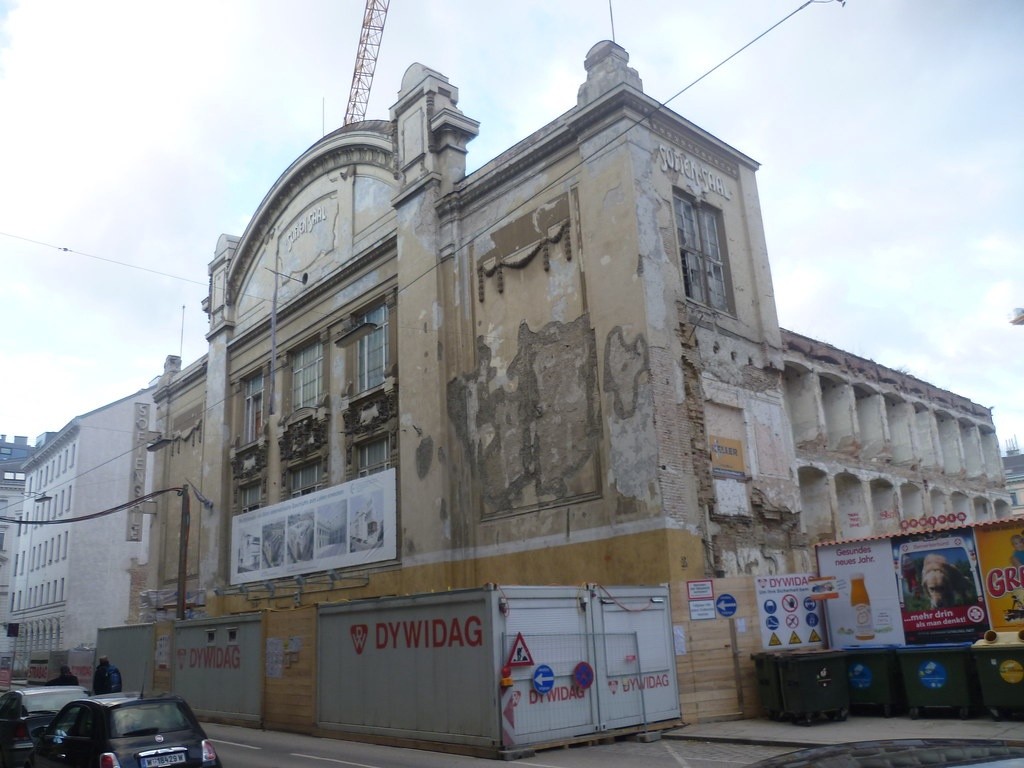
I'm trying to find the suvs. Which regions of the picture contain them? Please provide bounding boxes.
[0,685,91,768]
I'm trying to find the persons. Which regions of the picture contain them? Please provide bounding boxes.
[92,656,115,696]
[1010,534,1024,577]
[46,665,79,686]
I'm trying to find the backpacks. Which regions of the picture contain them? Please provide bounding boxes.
[99,667,121,694]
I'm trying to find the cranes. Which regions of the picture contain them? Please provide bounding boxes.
[342,0,390,127]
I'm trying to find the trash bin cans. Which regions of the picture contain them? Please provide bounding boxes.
[841,643,906,718]
[894,641,981,720]
[748,646,849,727]
[970,630,1024,721]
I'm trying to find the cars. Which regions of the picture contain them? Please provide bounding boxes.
[26,691,224,768]
